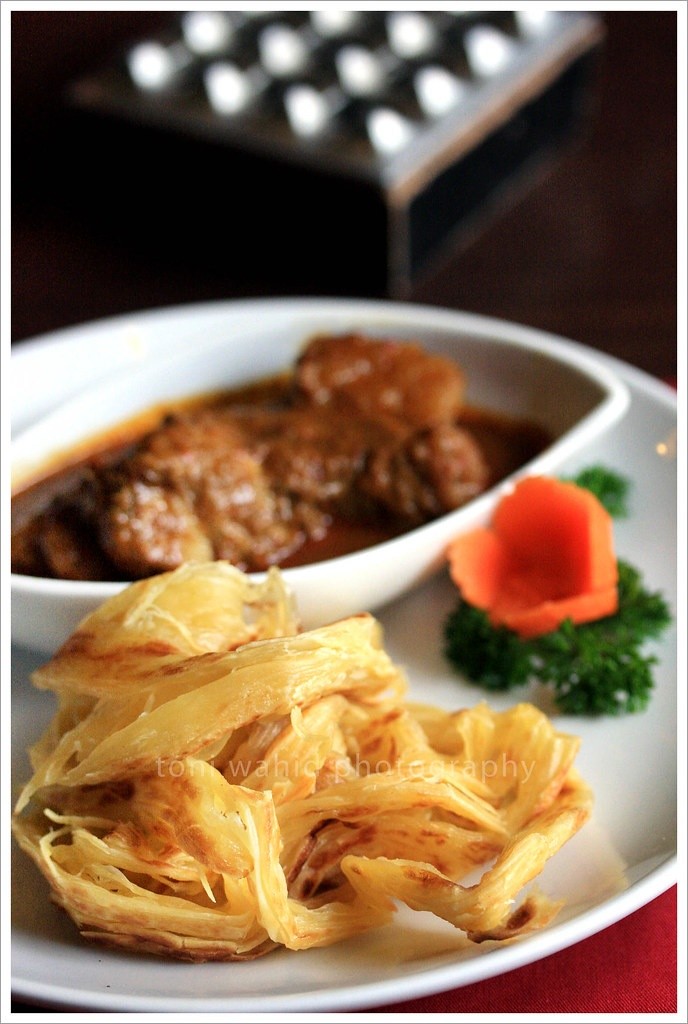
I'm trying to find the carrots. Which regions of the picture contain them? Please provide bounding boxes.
[444,474,619,638]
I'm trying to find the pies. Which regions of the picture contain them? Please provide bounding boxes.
[12,560,592,963]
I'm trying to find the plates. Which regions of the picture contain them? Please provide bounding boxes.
[12,293,678,1014]
[10,309,630,658]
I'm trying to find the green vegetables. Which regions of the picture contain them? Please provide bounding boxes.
[437,468,674,717]
